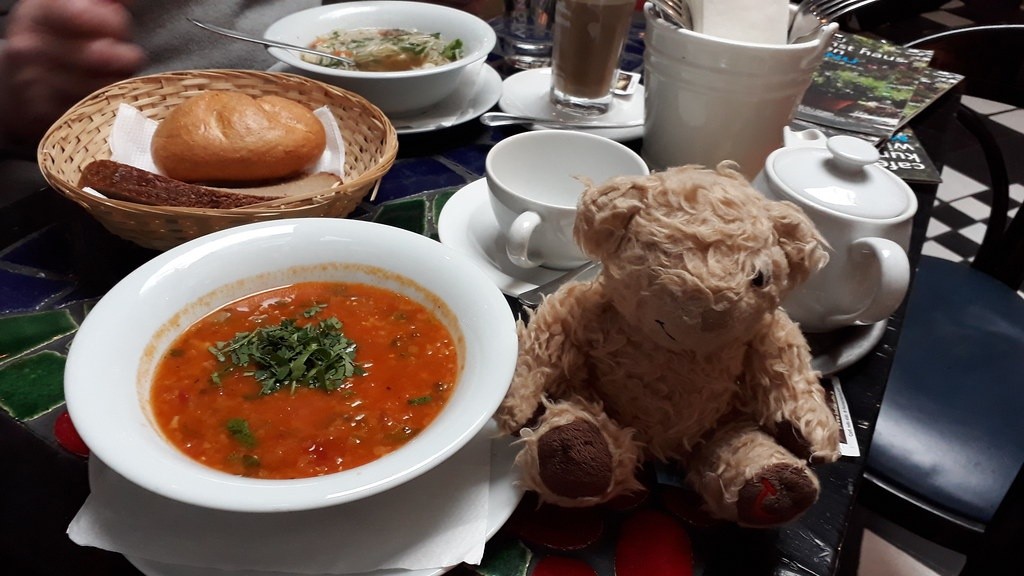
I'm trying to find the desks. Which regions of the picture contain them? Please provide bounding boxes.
[0,84,971,576]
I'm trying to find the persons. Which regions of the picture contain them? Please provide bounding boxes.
[0,1,325,134]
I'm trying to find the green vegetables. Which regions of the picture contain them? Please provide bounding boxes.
[322,30,463,68]
[208,302,430,466]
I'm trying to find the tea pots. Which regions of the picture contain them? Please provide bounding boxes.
[750,125,918,333]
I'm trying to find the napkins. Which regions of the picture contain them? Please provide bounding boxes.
[81,102,346,200]
[65,418,492,574]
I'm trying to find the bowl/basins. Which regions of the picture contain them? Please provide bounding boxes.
[263,0,493,112]
[64,217,518,511]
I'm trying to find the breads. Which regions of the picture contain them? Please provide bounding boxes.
[150,89,326,182]
[78,159,343,209]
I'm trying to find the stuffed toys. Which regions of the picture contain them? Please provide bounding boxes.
[489,160,841,527]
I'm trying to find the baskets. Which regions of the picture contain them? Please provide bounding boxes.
[36,67,399,251]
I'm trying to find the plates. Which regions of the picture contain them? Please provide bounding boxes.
[439,177,606,305]
[812,318,885,371]
[499,68,644,139]
[266,62,504,133]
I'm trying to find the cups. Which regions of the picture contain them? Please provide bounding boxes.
[644,0,839,183]
[503,0,557,68]
[550,0,636,118]
[486,129,649,270]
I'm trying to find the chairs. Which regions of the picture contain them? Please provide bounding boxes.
[855,252,1024,576]
[868,103,1024,576]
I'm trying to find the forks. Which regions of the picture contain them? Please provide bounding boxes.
[648,0,693,32]
[787,0,876,45]
